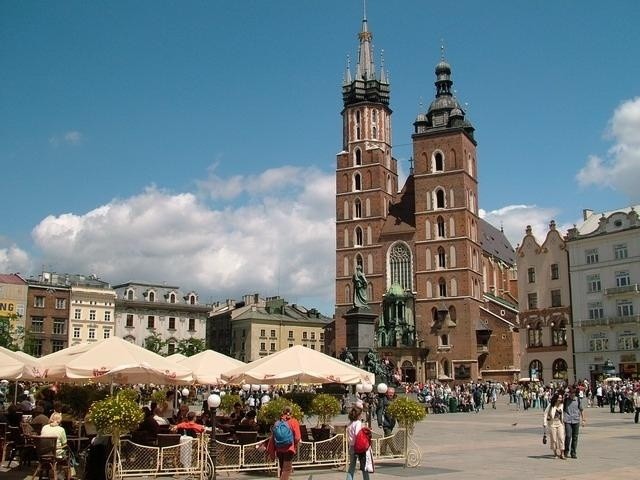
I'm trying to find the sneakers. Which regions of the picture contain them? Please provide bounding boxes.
[554,453,577,460]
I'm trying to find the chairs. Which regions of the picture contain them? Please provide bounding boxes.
[0,409,342,480]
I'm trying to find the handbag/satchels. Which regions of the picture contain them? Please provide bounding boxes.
[354,427,375,474]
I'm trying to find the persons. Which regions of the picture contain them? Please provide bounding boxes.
[543,392,568,460]
[402,376,640,425]
[351,264,370,310]
[562,388,586,459]
[0,379,403,479]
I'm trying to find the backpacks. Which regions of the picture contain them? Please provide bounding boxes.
[272,419,294,449]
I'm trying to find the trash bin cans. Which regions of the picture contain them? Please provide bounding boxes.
[449,397,457,413]
